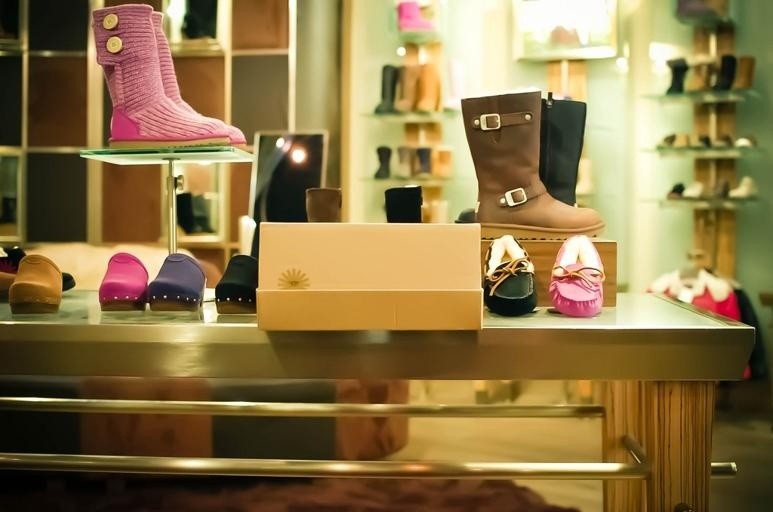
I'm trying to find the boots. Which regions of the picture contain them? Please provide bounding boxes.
[150,11,247,149]
[176,191,217,236]
[454,92,587,225]
[91,4,230,147]
[460,90,606,239]
[372,0,455,180]
[662,0,763,200]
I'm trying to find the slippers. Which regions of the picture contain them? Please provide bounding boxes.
[149,253,208,311]
[215,254,258,314]
[98,252,149,311]
[0,247,76,299]
[8,252,64,314]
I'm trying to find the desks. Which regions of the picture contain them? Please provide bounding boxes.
[0,288,757,512]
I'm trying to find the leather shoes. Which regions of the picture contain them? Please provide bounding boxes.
[483,234,537,317]
[548,233,606,317]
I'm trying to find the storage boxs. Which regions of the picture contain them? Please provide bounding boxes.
[256,220,484,330]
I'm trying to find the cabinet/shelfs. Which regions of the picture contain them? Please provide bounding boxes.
[642,15,760,211]
[358,32,457,188]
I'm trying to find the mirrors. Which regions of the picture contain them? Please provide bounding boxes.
[247,130,330,259]
